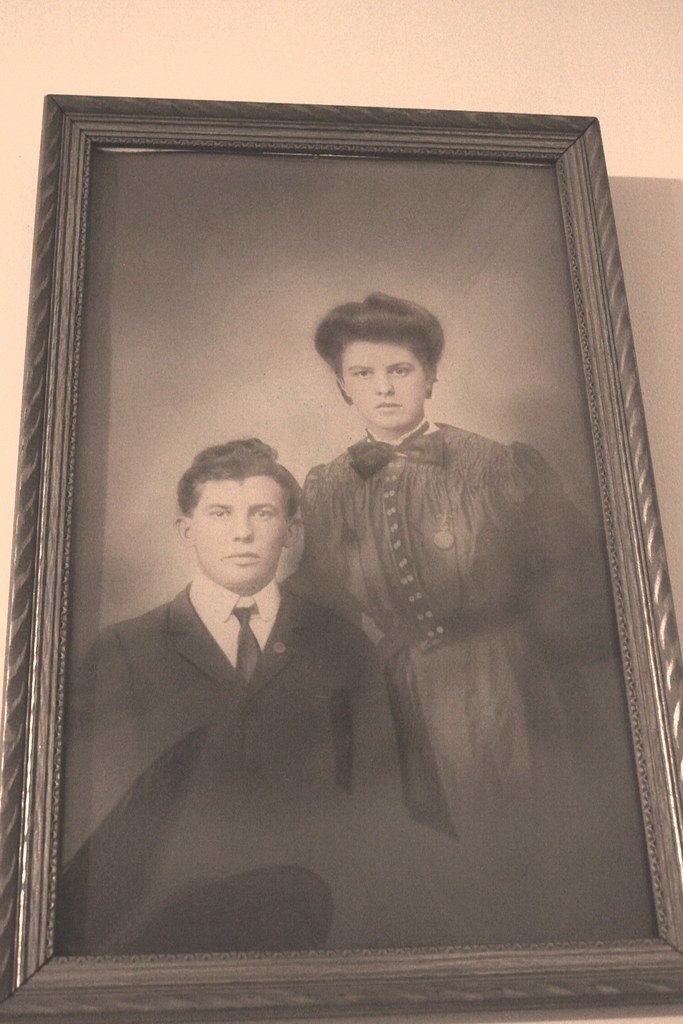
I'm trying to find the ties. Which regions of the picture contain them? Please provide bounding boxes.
[230,602,262,683]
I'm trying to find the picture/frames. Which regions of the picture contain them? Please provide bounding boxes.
[0,95,683,1023]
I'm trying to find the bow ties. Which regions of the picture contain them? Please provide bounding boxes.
[348,429,445,480]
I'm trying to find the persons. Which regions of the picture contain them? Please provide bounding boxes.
[279,295,619,834]
[54,441,459,957]
